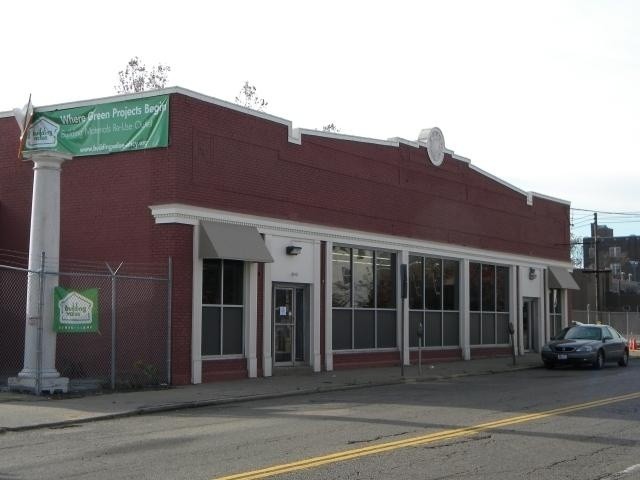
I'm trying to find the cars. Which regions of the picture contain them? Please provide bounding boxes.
[540,320,630,370]
